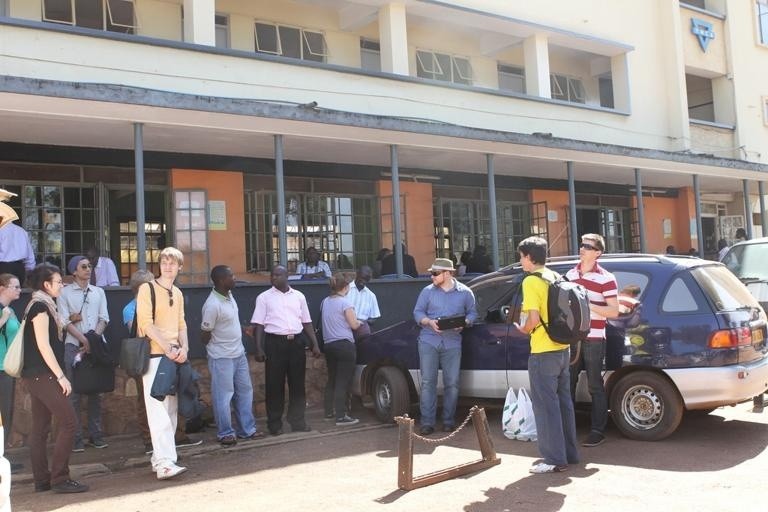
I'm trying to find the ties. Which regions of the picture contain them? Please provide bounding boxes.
[90,267,97,286]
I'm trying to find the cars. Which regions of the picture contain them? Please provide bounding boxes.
[715,237,767,314]
[354,253,767,442]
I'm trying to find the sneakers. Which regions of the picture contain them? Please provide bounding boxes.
[86,439,108,449]
[11,461,27,474]
[290,424,313,433]
[580,431,607,448]
[322,414,337,421]
[441,424,454,433]
[144,443,154,455]
[150,461,188,480]
[237,431,266,441]
[71,442,85,453]
[219,434,237,445]
[33,480,50,492]
[174,437,204,448]
[50,477,91,494]
[269,429,285,437]
[420,425,435,436]
[527,461,569,475]
[335,414,361,427]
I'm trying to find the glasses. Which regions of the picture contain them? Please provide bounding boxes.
[577,241,600,252]
[168,289,174,308]
[430,270,448,276]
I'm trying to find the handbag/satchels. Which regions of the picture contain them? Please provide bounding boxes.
[310,326,324,352]
[68,358,116,396]
[2,317,28,380]
[118,335,153,377]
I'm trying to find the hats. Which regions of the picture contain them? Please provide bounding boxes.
[426,257,457,272]
[67,255,88,274]
[329,270,356,291]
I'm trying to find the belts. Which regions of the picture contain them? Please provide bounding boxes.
[264,332,305,341]
[146,352,167,358]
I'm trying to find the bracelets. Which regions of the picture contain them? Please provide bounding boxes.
[427,320,431,325]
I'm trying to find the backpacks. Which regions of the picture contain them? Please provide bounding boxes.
[547,279,592,345]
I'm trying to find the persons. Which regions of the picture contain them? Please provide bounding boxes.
[1,225,204,493]
[666,245,674,254]
[295,247,331,279]
[412,258,477,435]
[689,248,696,255]
[459,246,493,275]
[372,243,418,279]
[201,264,380,447]
[565,233,620,447]
[736,228,749,241]
[719,236,726,250]
[603,284,641,370]
[513,236,580,475]
